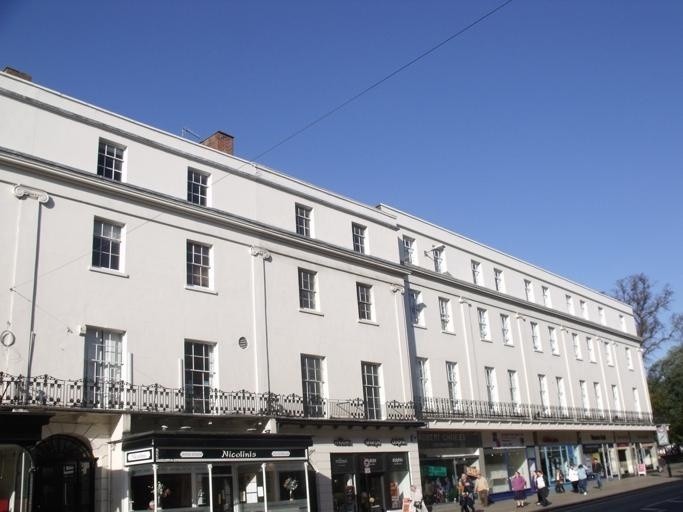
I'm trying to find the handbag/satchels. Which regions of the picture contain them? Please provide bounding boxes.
[414,501,422,509]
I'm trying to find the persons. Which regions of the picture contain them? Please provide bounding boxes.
[532,471,552,506]
[554,463,588,495]
[511,472,526,508]
[592,458,601,489]
[423,473,489,512]
